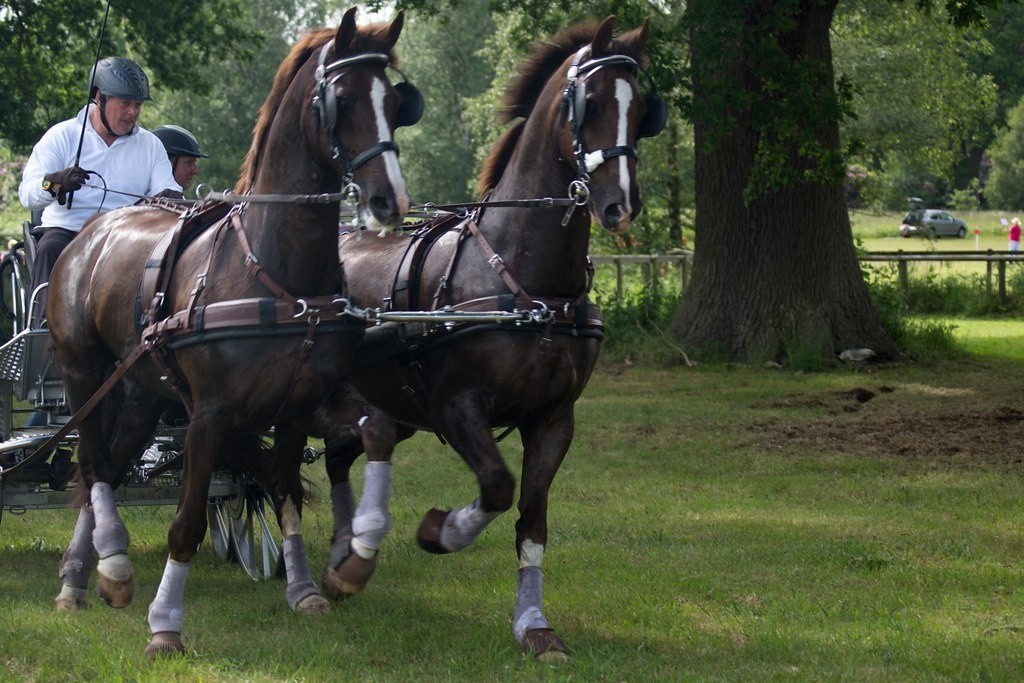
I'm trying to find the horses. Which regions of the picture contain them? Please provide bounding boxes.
[45,5,412,659]
[217,15,653,666]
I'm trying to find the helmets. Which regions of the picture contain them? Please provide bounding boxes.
[151,125,208,157]
[89,57,153,100]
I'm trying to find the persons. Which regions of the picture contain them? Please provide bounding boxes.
[148,124,208,192]
[1007,217,1022,264]
[18,55,184,330]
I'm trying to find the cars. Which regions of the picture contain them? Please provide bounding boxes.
[899,209,968,238]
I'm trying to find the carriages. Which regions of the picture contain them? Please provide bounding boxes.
[0,2,673,669]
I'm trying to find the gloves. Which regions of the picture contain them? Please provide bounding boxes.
[153,189,185,200]
[43,167,90,194]
